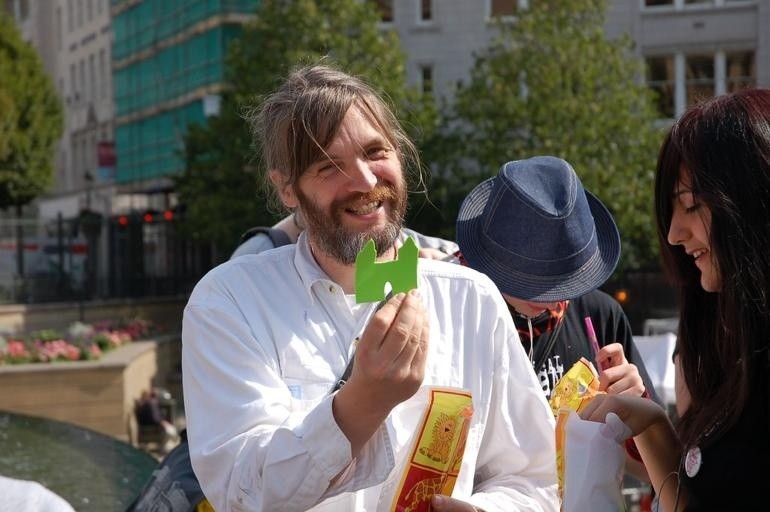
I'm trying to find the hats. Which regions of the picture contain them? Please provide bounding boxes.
[457,155,621,303]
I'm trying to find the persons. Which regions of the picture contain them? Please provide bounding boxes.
[456,155,667,412]
[182,67,561,512]
[580,90,770,512]
[229,213,460,264]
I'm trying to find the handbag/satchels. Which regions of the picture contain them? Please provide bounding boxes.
[128,441,218,512]
